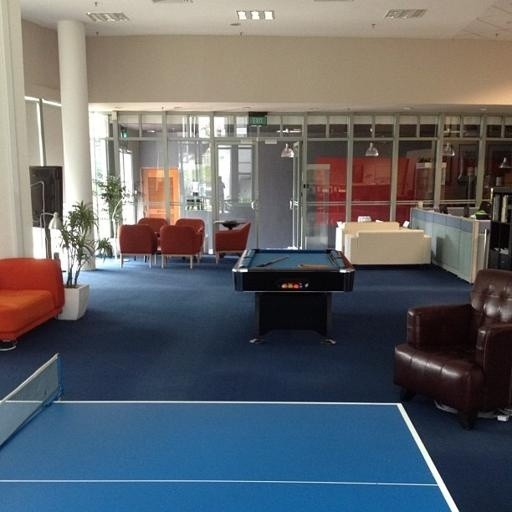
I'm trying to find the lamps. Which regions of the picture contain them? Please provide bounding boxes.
[498,158,512,168]
[365,143,379,156]
[40,212,63,230]
[442,143,456,156]
[280,128,295,157]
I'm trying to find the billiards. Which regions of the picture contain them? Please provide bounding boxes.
[281,282,309,289]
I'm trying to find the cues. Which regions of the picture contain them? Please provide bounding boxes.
[256,256,288,267]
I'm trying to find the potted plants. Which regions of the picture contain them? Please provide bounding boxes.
[92,171,135,256]
[54,201,113,321]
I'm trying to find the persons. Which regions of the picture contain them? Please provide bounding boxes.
[219,177,226,213]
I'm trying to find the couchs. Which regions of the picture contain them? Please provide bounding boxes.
[335,221,432,270]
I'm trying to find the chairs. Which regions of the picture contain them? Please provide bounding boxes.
[118,218,205,269]
[216,221,251,264]
[439,201,511,270]
[394,269,512,430]
[0,258,65,351]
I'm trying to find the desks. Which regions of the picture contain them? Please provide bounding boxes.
[232,249,355,345]
[0,352,461,512]
